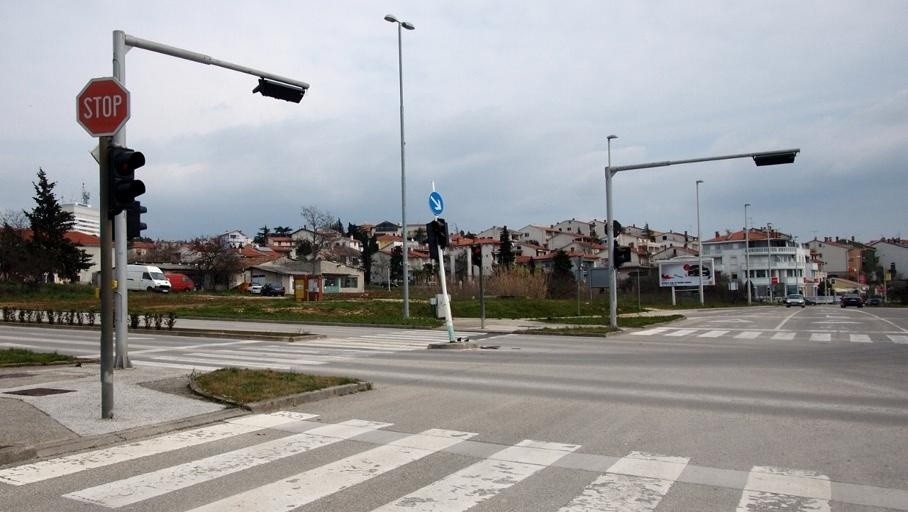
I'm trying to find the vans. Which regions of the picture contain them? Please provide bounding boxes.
[164,273,195,293]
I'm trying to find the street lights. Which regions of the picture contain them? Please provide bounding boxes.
[695,178,706,306]
[766,222,773,304]
[744,203,752,305]
[383,12,417,318]
[794,235,799,294]
[853,269,860,294]
[607,132,619,165]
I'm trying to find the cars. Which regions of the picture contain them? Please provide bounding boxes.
[688,264,711,277]
[840,293,864,308]
[246,282,263,294]
[782,296,817,307]
[865,294,884,307]
[261,281,286,297]
[786,293,806,308]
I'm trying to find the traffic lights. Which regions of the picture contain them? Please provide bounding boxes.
[127,199,147,239]
[470,244,482,267]
[431,217,448,249]
[102,144,148,221]
[614,239,626,269]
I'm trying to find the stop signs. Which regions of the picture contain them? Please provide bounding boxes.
[72,76,133,140]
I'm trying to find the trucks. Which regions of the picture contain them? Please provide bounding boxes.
[127,265,172,294]
